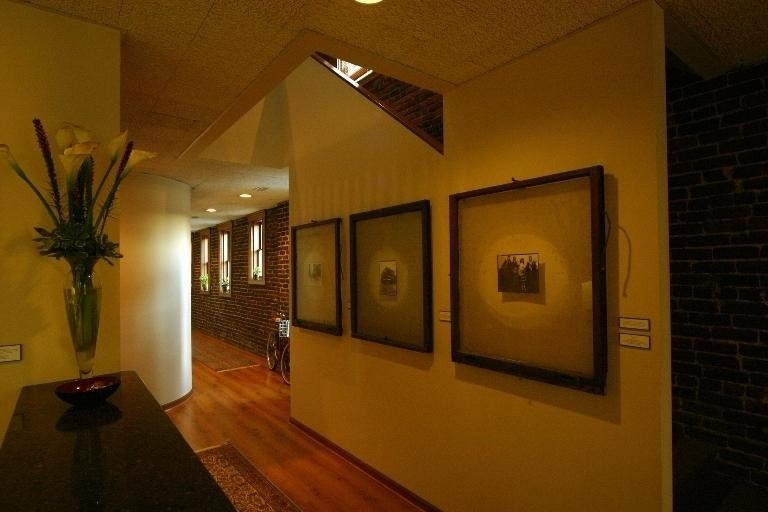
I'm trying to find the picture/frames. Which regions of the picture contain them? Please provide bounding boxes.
[289,216,345,339]
[348,196,437,355]
[447,164,619,398]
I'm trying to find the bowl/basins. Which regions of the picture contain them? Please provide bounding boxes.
[53,375,121,407]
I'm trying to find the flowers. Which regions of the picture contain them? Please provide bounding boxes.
[0,114,158,360]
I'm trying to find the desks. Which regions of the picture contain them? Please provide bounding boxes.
[0,371,237,511]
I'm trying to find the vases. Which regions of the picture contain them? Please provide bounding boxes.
[62,254,103,381]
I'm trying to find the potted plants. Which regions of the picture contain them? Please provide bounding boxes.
[217,276,230,295]
[251,266,262,282]
[198,273,209,292]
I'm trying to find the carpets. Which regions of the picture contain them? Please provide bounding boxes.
[193,438,304,512]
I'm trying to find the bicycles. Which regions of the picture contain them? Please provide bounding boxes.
[266,312,290,385]
